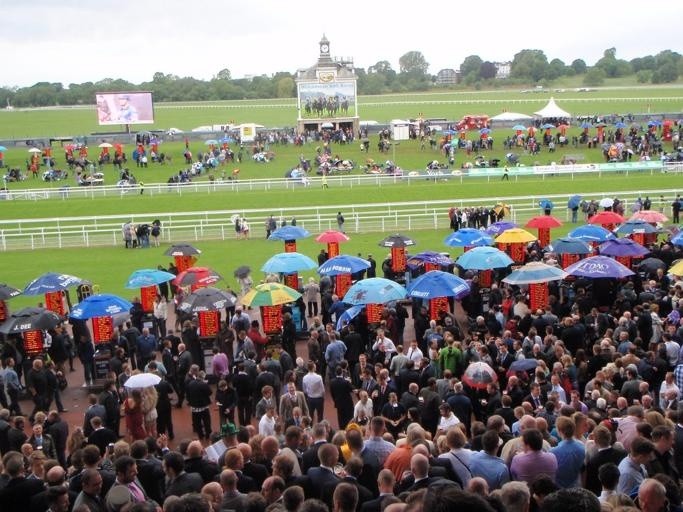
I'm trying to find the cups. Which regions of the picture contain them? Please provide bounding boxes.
[586,390,591,397]
[107,442,114,455]
[632,399,638,404]
[502,390,507,395]
[333,462,342,475]
[537,405,544,409]
[417,397,424,403]
[480,398,486,406]
[35,444,42,451]
[660,391,665,397]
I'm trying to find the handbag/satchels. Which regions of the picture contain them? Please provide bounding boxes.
[59,379,68,390]
[119,404,126,417]
[145,408,158,421]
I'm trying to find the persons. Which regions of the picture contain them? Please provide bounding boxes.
[114,94,137,124]
[1,114,683,196]
[2,194,683,510]
[96,94,113,124]
[305,92,352,115]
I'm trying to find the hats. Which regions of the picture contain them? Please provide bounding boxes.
[219,423,241,437]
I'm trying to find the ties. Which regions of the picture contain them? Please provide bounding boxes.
[535,398,540,406]
[128,484,144,501]
